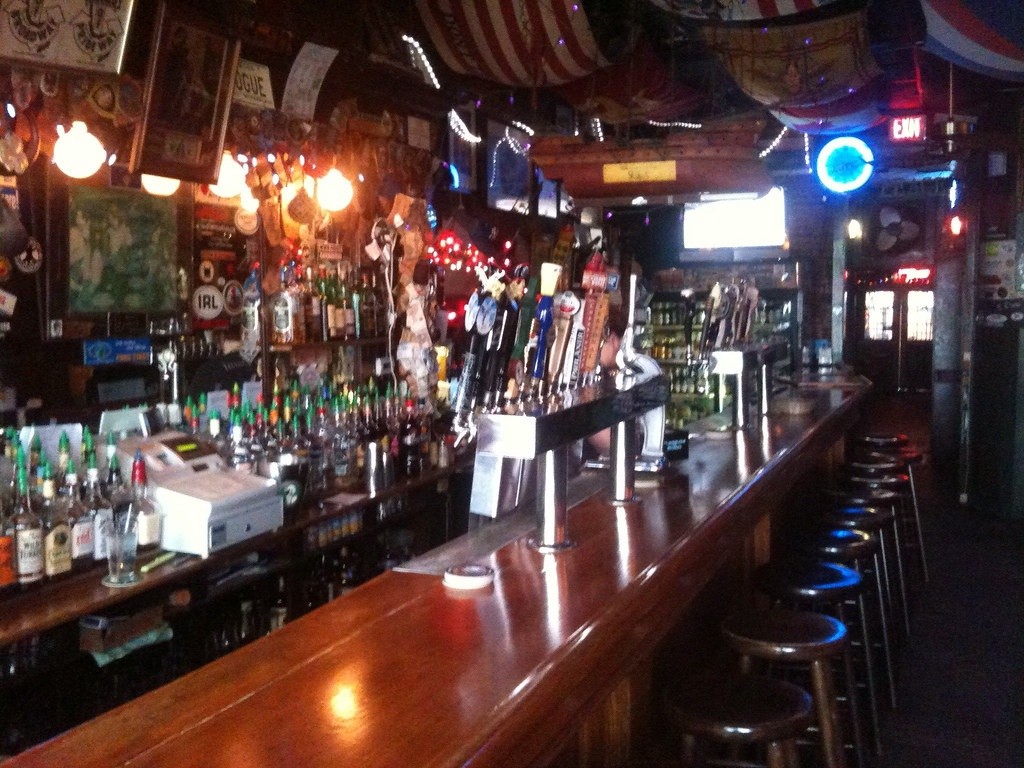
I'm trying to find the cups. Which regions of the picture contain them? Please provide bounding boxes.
[103,520,137,583]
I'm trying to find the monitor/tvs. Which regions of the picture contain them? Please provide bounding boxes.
[677,184,793,264]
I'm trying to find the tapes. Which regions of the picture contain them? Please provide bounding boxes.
[442,563,495,589]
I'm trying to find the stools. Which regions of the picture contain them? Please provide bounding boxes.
[668,432,926,768]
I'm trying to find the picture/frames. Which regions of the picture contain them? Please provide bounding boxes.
[447,93,559,220]
[127,0,242,184]
[0,0,138,76]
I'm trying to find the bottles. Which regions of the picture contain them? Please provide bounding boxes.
[1,426,413,761]
[649,300,791,434]
[154,261,452,519]
